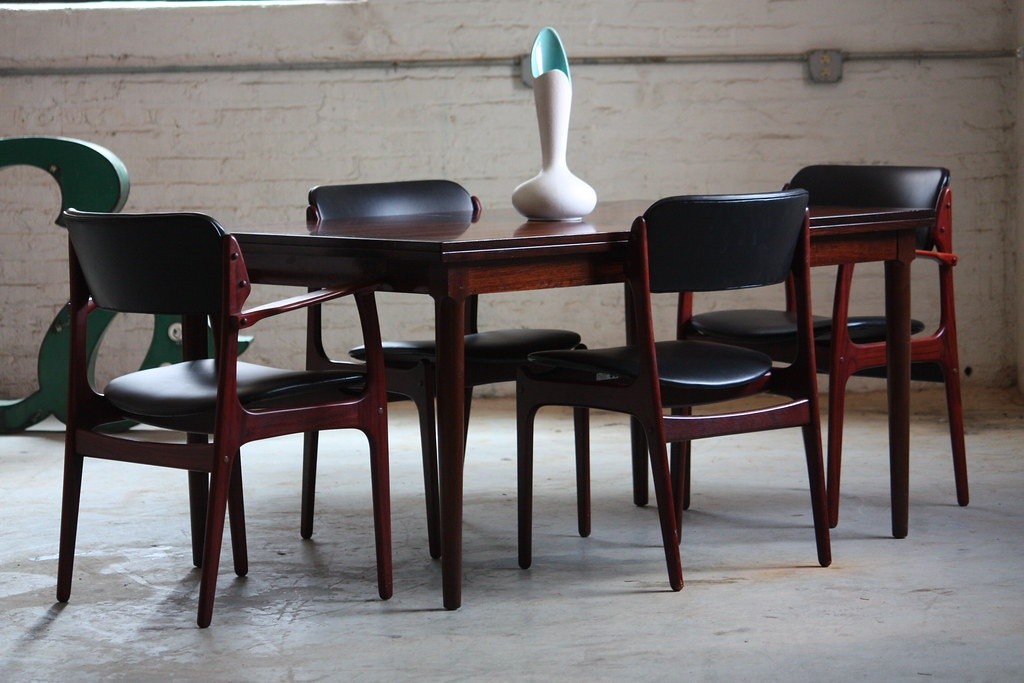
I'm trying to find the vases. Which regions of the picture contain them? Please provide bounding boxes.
[513,26,599,222]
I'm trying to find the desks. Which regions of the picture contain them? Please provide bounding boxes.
[184,205,945,611]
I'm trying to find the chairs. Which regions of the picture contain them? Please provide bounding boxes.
[514,187,833,594]
[682,164,972,531]
[297,178,593,562]
[54,207,396,605]
